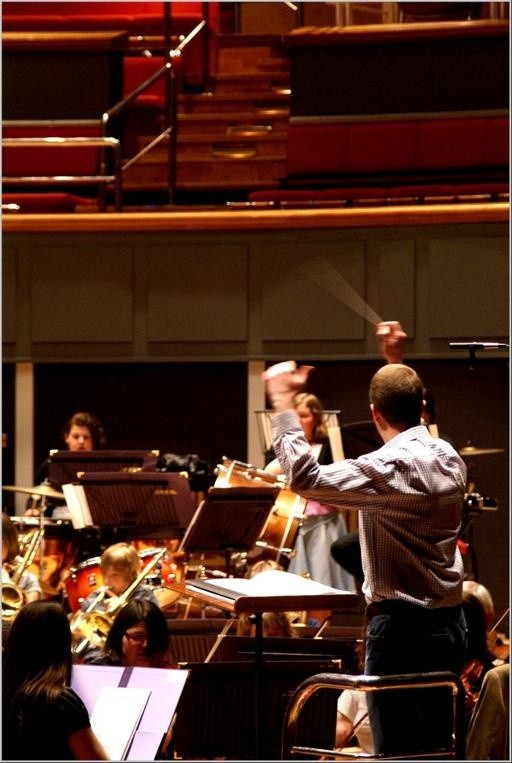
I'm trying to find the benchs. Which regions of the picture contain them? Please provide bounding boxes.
[2,2,221,208]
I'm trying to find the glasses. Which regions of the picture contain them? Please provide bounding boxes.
[125,632,145,641]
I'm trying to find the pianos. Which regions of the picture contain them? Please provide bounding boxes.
[8,515,98,540]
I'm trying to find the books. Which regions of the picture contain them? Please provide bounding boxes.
[185,569,355,613]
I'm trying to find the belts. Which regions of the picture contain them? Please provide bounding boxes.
[365,598,408,621]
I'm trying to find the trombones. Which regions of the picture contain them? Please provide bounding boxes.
[1,528,45,620]
[68,547,169,656]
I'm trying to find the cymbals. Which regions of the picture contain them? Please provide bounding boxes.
[2,482,65,499]
[457,446,505,457]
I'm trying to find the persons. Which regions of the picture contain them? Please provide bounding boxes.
[264,392,346,590]
[2,512,43,605]
[2,599,111,759]
[320,644,375,760]
[78,542,170,666]
[25,413,124,564]
[262,321,468,760]
[461,580,509,759]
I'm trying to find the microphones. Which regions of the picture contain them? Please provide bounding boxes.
[449,342,507,351]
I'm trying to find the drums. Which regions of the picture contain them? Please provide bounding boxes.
[63,556,103,613]
[214,457,310,551]
[137,548,185,612]
